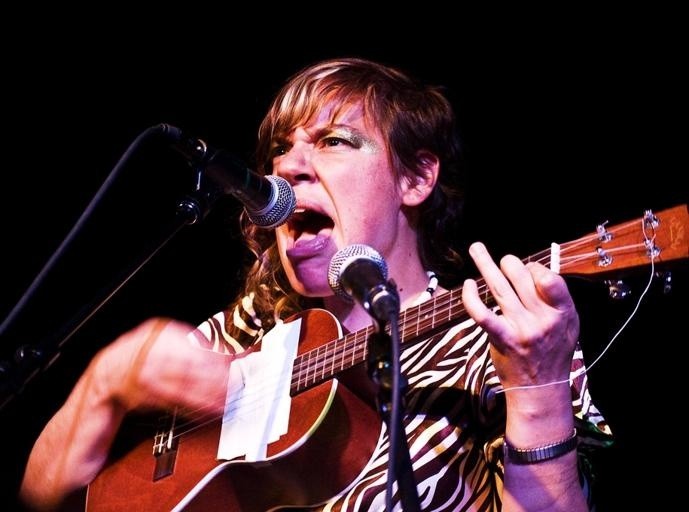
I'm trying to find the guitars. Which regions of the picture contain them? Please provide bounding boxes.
[84,206,688,511]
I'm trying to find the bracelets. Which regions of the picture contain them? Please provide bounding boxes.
[498,429,583,471]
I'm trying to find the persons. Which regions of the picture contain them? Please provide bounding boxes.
[17,56,593,511]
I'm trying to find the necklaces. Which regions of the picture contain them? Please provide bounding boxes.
[407,266,443,305]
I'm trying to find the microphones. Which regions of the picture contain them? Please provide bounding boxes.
[158,121,298,230]
[328,243,401,323]
